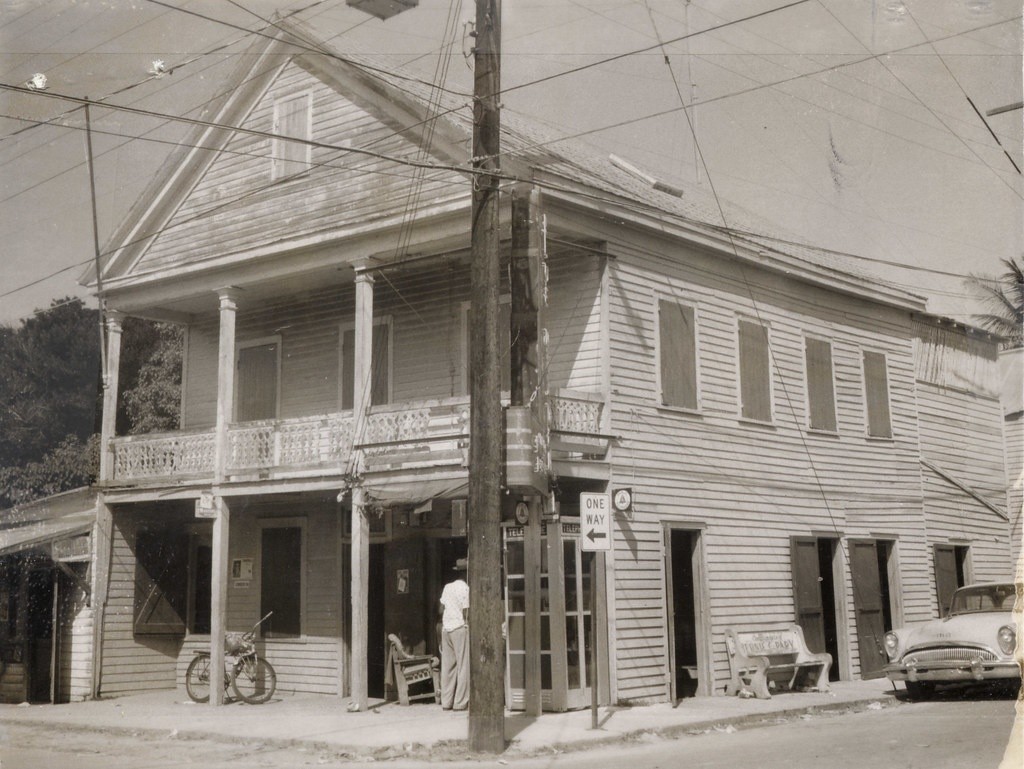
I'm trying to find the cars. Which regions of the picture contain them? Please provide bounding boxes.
[883,581,1020,703]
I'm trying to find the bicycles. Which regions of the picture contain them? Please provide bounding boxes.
[185,628,277,705]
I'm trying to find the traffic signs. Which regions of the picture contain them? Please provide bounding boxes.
[579,492,612,552]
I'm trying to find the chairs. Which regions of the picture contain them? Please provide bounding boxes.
[388,633,441,706]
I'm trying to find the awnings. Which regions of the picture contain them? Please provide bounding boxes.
[0,513,96,606]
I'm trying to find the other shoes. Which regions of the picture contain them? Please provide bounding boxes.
[443,708,453,710]
[453,707,468,712]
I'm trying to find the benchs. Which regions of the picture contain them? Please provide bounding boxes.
[724,625,833,699]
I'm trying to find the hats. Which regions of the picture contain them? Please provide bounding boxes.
[453,558,468,570]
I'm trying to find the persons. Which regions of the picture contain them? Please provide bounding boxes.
[439,558,469,711]
